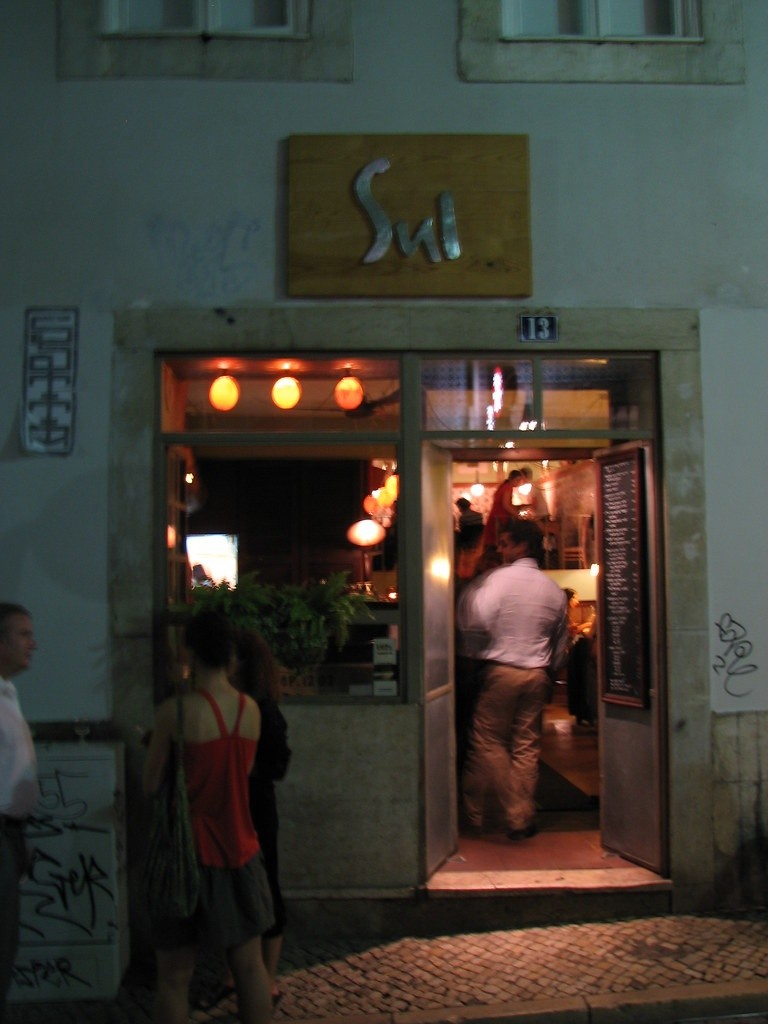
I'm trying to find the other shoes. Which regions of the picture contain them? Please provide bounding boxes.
[506,823,544,842]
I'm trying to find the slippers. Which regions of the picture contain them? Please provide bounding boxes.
[188,973,236,1011]
[269,989,285,1006]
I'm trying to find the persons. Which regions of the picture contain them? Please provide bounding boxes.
[1,602,42,1005]
[139,607,276,1024]
[485,470,528,555]
[455,518,565,838]
[188,632,293,1007]
[452,525,488,596]
[454,498,484,531]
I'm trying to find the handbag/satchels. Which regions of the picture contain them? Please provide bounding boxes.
[121,693,202,921]
